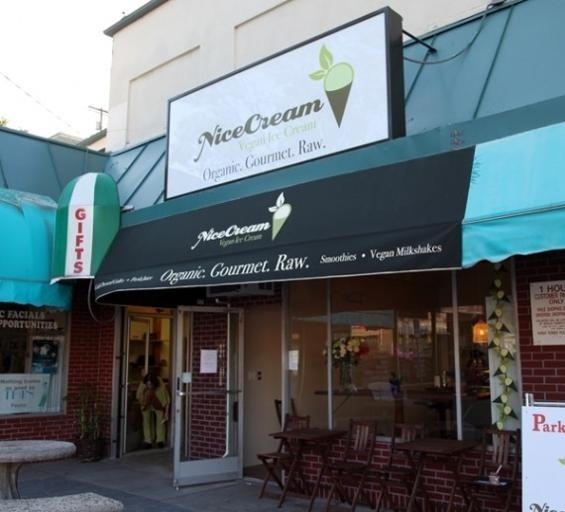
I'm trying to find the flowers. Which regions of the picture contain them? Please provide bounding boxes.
[323,334,368,360]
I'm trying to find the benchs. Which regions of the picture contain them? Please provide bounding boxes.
[0,491,123,512]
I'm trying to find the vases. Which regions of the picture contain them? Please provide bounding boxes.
[339,358,352,392]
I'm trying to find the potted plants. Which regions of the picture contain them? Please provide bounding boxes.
[62,383,99,459]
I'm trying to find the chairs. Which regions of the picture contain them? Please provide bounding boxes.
[255,413,521,512]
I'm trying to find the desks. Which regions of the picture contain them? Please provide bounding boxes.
[0,439,77,499]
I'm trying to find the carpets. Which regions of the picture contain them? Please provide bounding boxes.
[17,475,193,512]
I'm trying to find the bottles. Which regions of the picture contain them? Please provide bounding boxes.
[433,370,448,388]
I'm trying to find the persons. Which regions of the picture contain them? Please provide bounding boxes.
[135,372,171,450]
